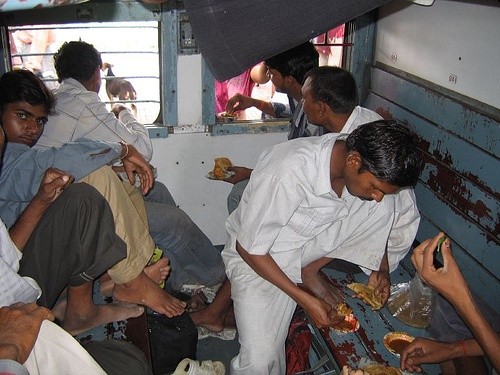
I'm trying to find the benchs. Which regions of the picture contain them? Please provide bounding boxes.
[303,62,500,375]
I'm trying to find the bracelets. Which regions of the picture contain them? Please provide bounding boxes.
[119,140,129,161]
[256,100,266,110]
[460,338,466,362]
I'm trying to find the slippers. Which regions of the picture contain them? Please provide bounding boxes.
[171,357,226,375]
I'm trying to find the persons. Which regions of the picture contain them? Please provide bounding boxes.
[221,117,430,375]
[0,121,150,375]
[400,232,500,375]
[214,62,272,122]
[186,66,425,332]
[314,24,345,68]
[12,24,65,91]
[1,69,186,318]
[184,40,335,315]
[29,38,228,305]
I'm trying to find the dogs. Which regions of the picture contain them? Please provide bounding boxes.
[102,63,136,120]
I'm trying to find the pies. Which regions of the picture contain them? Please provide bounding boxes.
[346,282,383,310]
